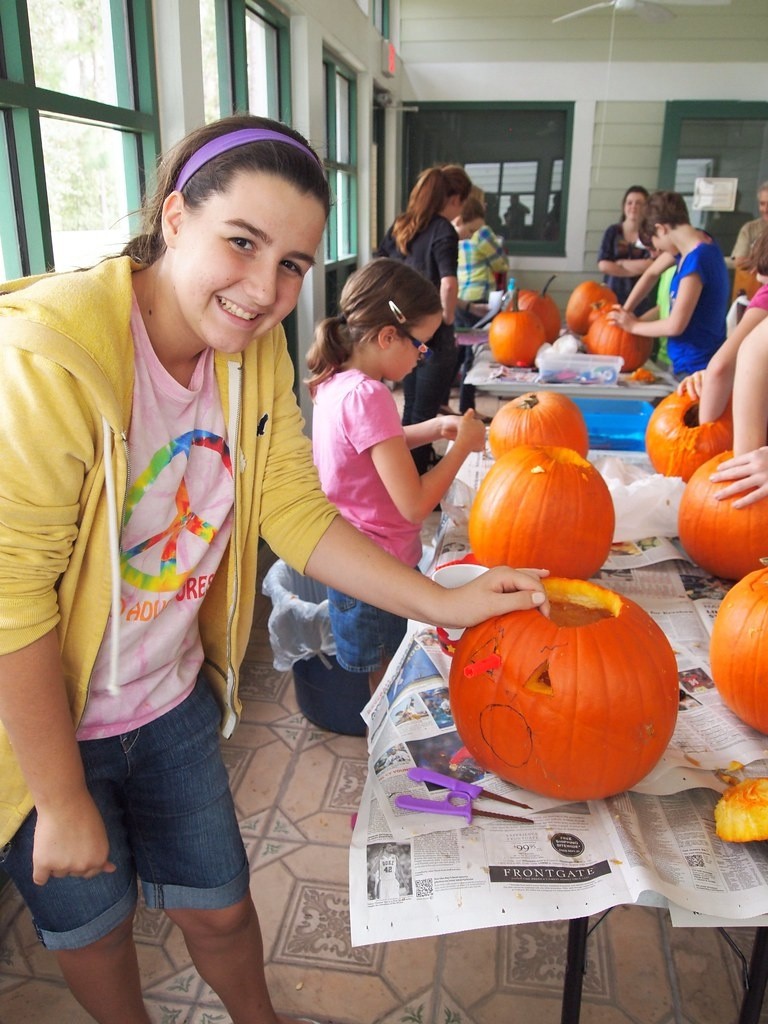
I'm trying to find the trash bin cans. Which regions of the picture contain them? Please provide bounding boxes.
[265,558,370,736]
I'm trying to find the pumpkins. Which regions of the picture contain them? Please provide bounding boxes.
[644,386,733,482]
[489,275,561,367]
[468,444,616,583]
[565,281,653,372]
[709,557,768,737]
[677,449,768,581]
[490,391,588,461]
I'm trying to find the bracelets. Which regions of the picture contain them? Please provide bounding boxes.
[466,302,471,313]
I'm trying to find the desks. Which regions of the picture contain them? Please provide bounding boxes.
[452,327,678,404]
[365,418,768,1024]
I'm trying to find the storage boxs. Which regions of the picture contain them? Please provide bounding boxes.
[535,352,625,386]
[571,398,655,452]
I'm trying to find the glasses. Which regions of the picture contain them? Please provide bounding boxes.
[399,328,434,365]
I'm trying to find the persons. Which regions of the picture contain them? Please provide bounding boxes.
[377,164,474,474]
[452,186,511,425]
[597,185,657,316]
[676,228,768,508]
[307,259,484,692]
[605,193,727,384]
[638,219,676,377]
[731,181,768,270]
[1,117,550,1024]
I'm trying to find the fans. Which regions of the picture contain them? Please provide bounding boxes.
[552,0,732,23]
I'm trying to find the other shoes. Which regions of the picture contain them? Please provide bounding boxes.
[479,415,493,425]
[430,453,444,465]
[437,406,461,417]
[432,502,443,512]
